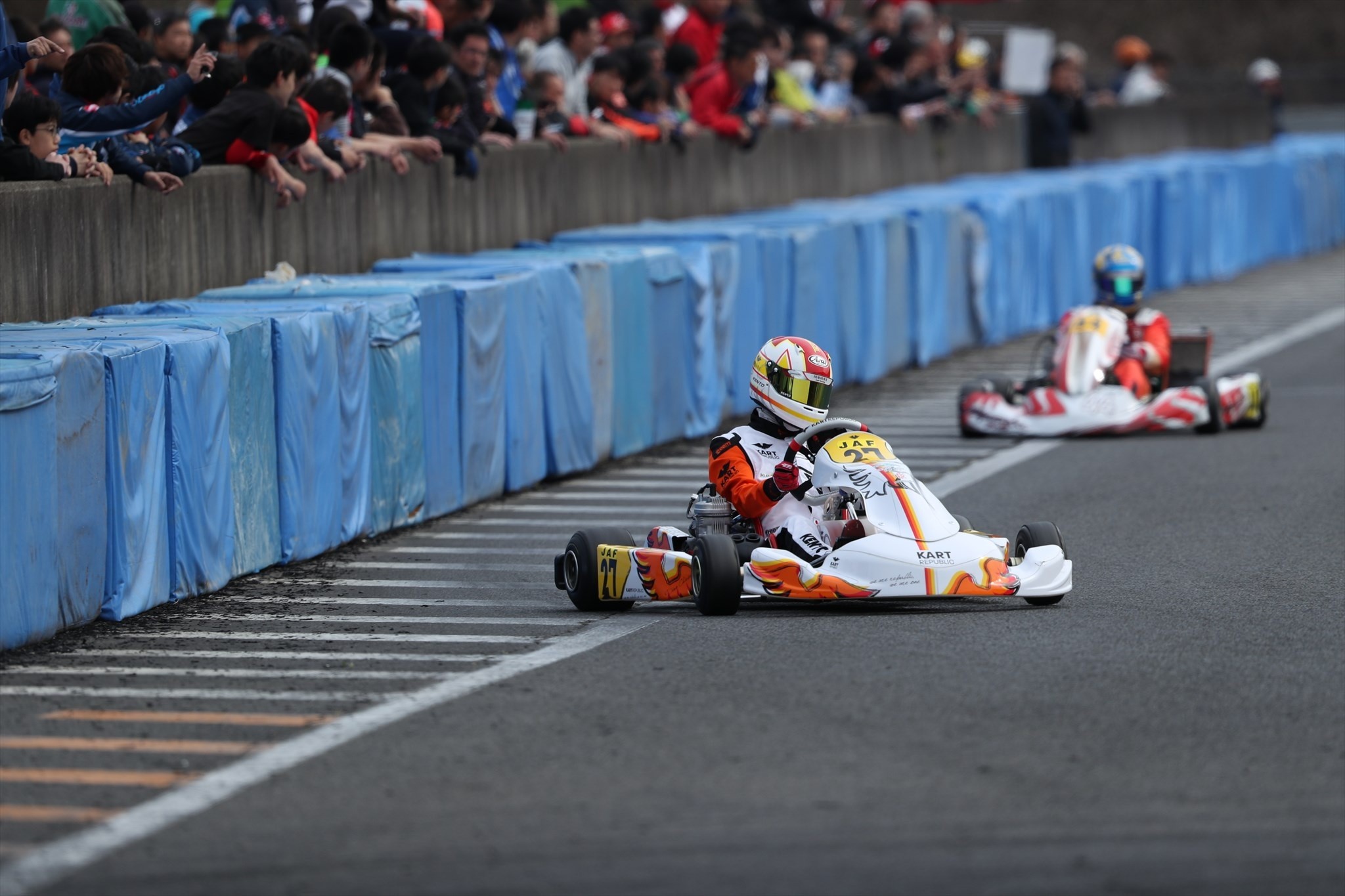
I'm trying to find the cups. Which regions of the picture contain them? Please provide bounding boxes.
[513,110,535,140]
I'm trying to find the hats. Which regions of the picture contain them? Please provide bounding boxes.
[601,12,630,35]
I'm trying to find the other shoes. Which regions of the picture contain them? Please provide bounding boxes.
[841,519,866,538]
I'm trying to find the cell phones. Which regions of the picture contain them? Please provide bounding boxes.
[201,51,218,74]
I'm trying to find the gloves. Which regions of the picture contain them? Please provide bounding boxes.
[763,461,799,502]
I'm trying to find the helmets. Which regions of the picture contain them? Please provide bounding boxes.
[749,336,834,430]
[1092,243,1145,311]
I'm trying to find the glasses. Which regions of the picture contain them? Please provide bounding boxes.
[38,126,60,138]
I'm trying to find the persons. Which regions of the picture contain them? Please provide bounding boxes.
[1021,242,1171,400]
[0,0,1284,205]
[708,336,866,569]
